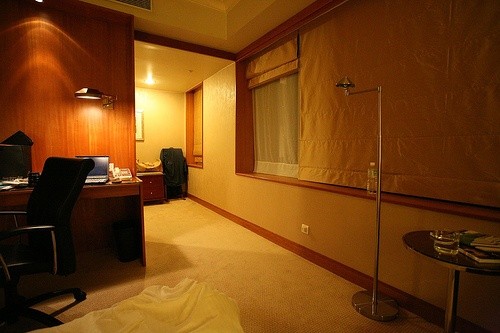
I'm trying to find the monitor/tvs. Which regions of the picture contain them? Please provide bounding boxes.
[0,145,32,181]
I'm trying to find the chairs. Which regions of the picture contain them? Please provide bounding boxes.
[0,156,96,327]
[160,148,189,199]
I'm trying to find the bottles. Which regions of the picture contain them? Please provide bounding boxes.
[367,162,377,194]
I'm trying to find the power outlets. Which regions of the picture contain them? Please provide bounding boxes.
[301,224,309,235]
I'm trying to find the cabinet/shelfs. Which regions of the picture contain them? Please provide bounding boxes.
[137,172,166,203]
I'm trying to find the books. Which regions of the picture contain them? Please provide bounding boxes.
[457,228,500,264]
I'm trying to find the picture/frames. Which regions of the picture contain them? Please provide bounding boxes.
[136,111,145,142]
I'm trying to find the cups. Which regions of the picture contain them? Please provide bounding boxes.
[433,227,460,256]
[28,172,40,187]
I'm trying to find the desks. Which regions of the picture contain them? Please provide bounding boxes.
[0,175,147,273]
[401,229,500,333]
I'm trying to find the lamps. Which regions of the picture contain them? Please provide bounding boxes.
[75,87,118,109]
[335,76,400,322]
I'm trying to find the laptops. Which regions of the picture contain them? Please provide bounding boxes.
[76,156,109,184]
